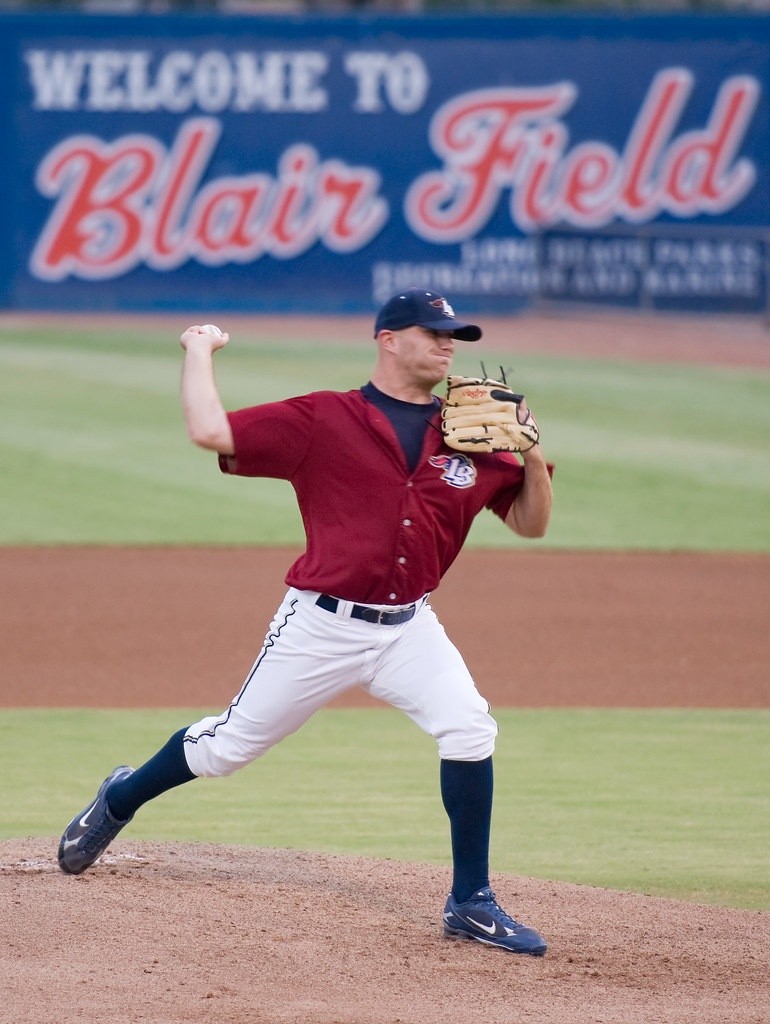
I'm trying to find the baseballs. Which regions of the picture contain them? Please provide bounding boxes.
[202,324,222,337]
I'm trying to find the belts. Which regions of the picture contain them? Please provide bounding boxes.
[317,594,418,627]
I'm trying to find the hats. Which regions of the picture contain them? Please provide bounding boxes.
[375,288,482,342]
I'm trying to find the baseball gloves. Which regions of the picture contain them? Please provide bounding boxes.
[440,374,540,453]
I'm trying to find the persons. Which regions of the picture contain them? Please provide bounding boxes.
[56,289,554,958]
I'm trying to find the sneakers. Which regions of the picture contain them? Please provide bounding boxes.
[440,886,549,961]
[58,766,140,877]
